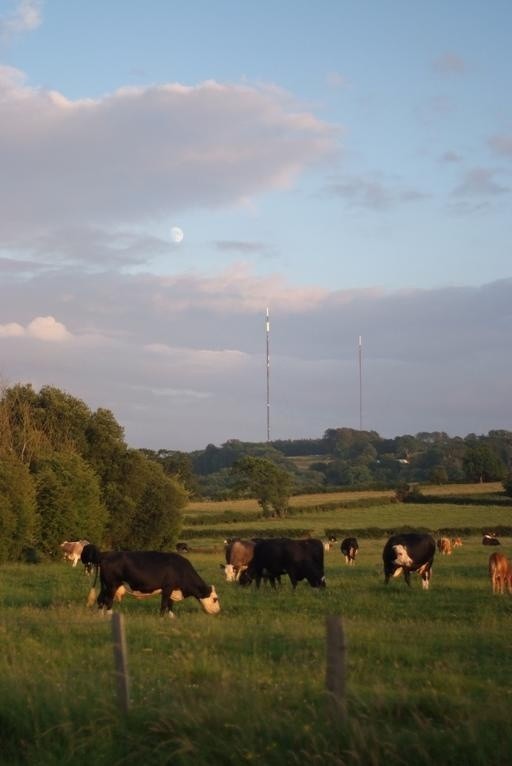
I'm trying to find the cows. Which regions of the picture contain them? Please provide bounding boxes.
[220,536,338,591]
[340,538,359,567]
[176,542,192,553]
[453,536,464,547]
[437,537,452,556]
[383,531,436,590]
[81,544,101,580]
[482,528,500,545]
[58,540,90,568]
[489,551,512,595]
[86,550,221,625]
